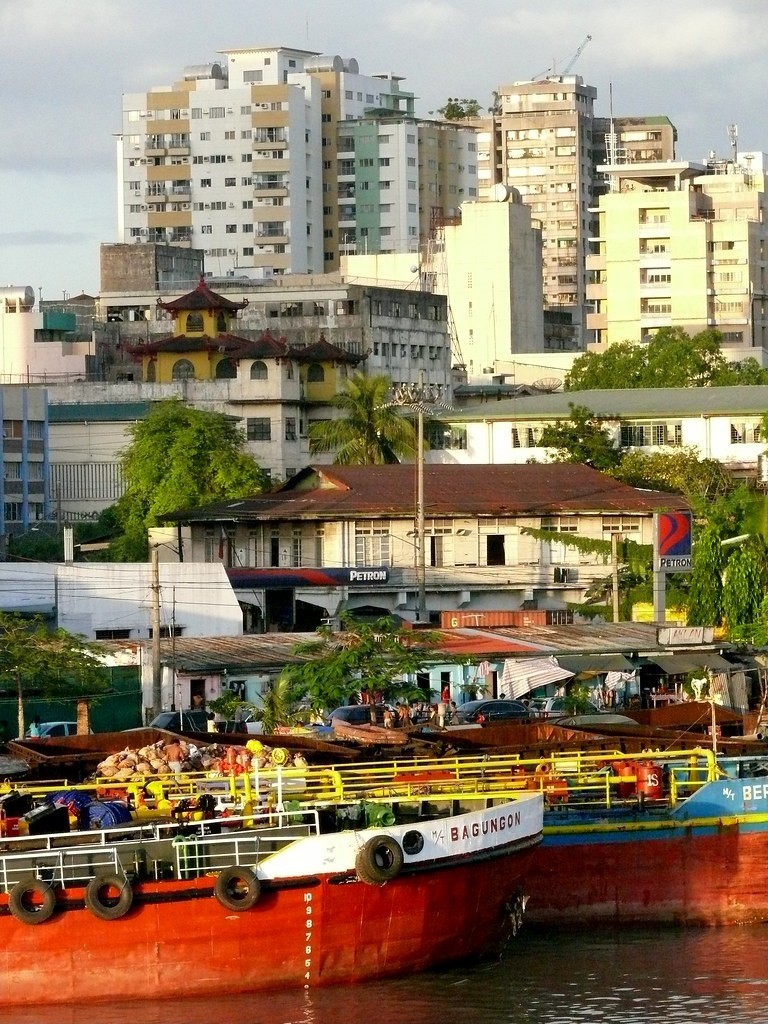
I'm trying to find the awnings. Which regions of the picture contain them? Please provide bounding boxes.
[558,654,635,671]
[649,652,736,675]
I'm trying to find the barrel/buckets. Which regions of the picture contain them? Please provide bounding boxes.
[637,767,663,805]
[618,767,637,798]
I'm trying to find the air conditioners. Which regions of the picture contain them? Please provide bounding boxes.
[402,351,406,356]
[141,158,146,164]
[429,353,438,359]
[184,203,190,209]
[262,151,269,157]
[229,249,235,254]
[261,103,268,110]
[411,352,419,358]
[140,229,147,236]
[204,249,210,255]
[141,205,147,211]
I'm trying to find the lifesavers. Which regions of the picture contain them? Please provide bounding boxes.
[215,865,260,911]
[354,835,405,884]
[8,879,55,925]
[85,872,133,921]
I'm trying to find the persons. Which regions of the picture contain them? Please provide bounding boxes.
[207,713,217,732]
[383,707,392,729]
[450,701,459,725]
[396,699,440,725]
[360,688,367,704]
[29,715,41,738]
[443,685,452,705]
[165,739,184,779]
[374,690,382,703]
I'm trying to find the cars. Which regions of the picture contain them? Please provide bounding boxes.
[302,704,401,735]
[526,696,612,720]
[10,721,94,742]
[444,699,536,727]
[226,707,269,736]
[147,708,209,733]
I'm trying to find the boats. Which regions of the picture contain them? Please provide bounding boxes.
[0,745,546,1009]
[321,744,768,935]
